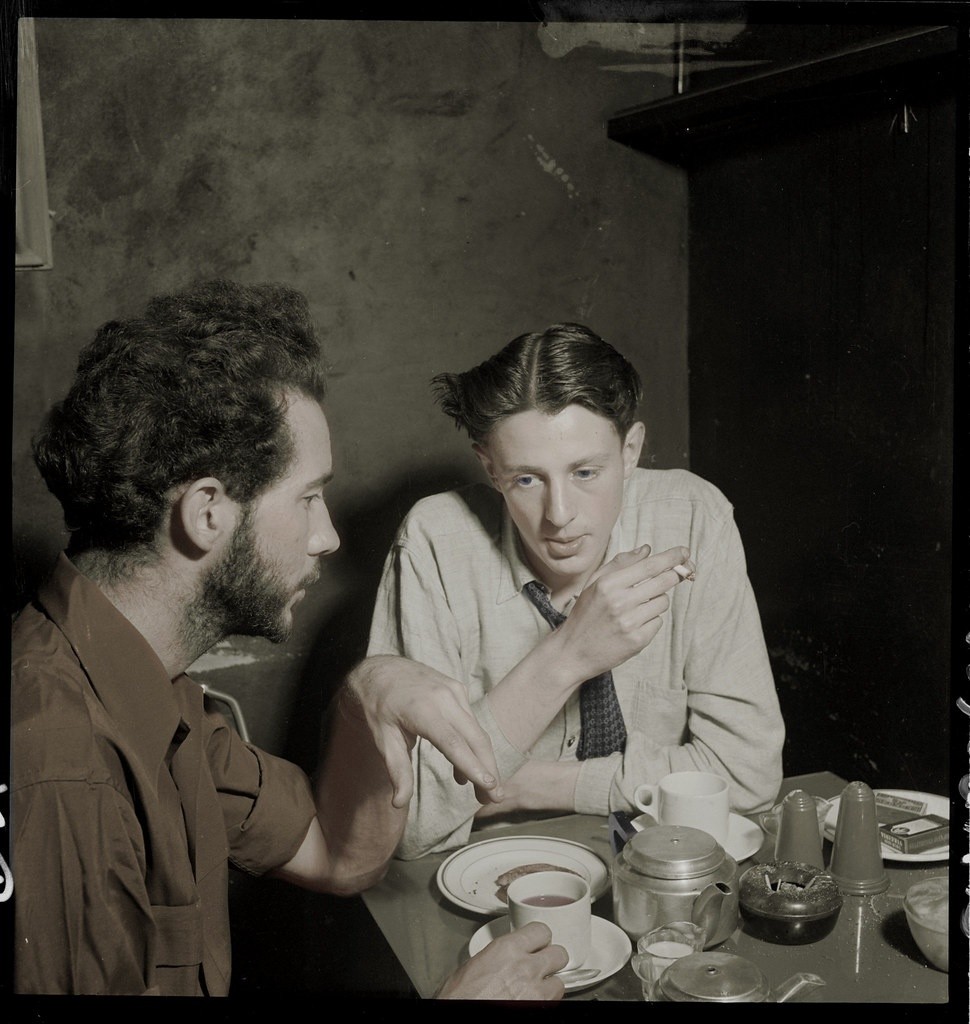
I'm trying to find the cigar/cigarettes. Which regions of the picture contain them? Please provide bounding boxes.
[672,564,696,583]
[764,874,815,891]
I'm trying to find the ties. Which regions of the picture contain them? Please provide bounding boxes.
[523,583,627,763]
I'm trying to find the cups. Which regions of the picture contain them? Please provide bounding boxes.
[631,920,708,999]
[633,770,729,847]
[507,870,591,972]
[752,797,835,868]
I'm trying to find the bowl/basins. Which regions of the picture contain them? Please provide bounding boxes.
[904,876,949,972]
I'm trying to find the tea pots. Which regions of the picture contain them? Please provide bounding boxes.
[648,952,826,1002]
[608,811,740,952]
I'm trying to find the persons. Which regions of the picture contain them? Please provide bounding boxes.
[366,324,786,860]
[10,281,569,1000]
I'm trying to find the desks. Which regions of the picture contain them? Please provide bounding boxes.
[361,767,948,1002]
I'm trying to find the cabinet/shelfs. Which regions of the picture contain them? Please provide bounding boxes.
[605,20,958,802]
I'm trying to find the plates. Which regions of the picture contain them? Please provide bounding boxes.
[436,836,612,917]
[469,915,633,994]
[630,812,763,865]
[824,789,950,862]
